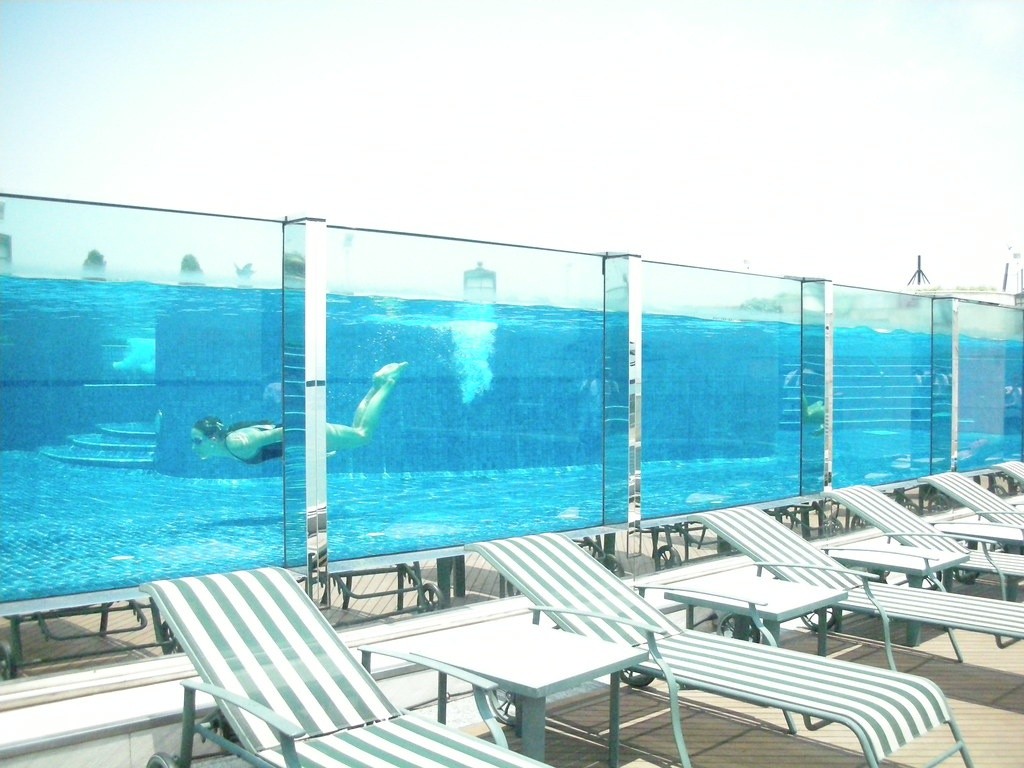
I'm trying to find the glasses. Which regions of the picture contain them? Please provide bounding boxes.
[191,437,202,445]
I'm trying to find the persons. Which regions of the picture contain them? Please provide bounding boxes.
[191,362,408,465]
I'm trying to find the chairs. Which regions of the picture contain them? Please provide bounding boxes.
[137,461,1024,768]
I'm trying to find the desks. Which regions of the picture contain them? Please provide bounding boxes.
[941,521,1024,603]
[664,572,848,656]
[410,618,650,762]
[831,542,970,647]
